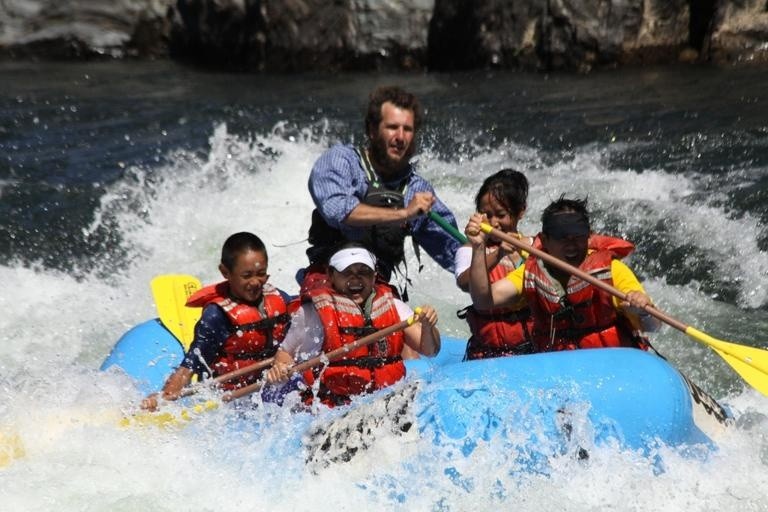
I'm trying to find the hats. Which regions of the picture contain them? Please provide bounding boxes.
[329,247,377,273]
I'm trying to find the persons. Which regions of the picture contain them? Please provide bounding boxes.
[454,169,535,360]
[141,232,293,418]
[465,194,661,351]
[295,87,463,361]
[258,242,440,416]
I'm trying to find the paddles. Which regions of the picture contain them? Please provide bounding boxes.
[121,307,421,429]
[479,221,768,396]
[151,273,204,385]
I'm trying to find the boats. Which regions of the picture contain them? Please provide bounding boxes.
[96,297,697,503]
